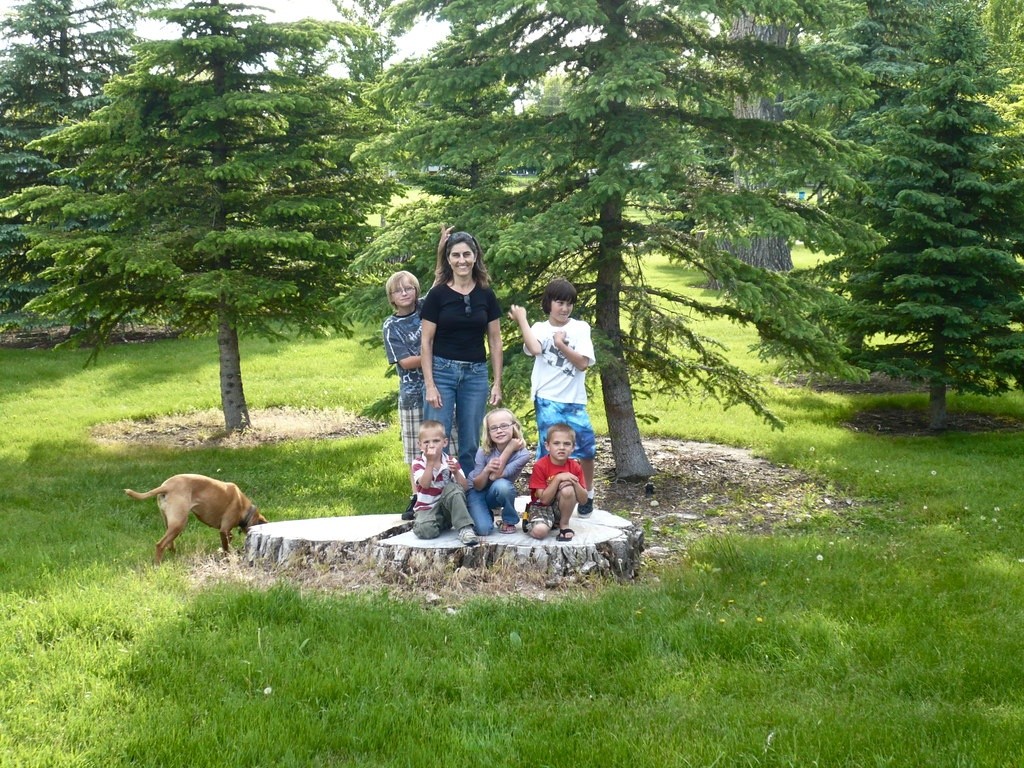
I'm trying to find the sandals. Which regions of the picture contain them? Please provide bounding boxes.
[500,522,516,534]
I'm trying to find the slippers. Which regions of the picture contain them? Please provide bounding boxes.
[557,529,574,541]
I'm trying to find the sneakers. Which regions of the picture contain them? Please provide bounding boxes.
[401,494,418,520]
[457,525,478,544]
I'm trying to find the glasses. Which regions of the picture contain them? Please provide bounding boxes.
[390,286,415,295]
[488,423,514,432]
[460,294,472,317]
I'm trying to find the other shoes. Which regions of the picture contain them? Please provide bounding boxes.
[578,497,595,517]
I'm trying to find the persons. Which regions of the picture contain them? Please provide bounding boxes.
[508,280,595,517]
[466,408,530,535]
[383,224,457,520]
[522,426,588,540]
[413,420,478,544]
[420,232,502,481]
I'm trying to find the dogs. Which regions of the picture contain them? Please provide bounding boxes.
[124,473,269,564]
